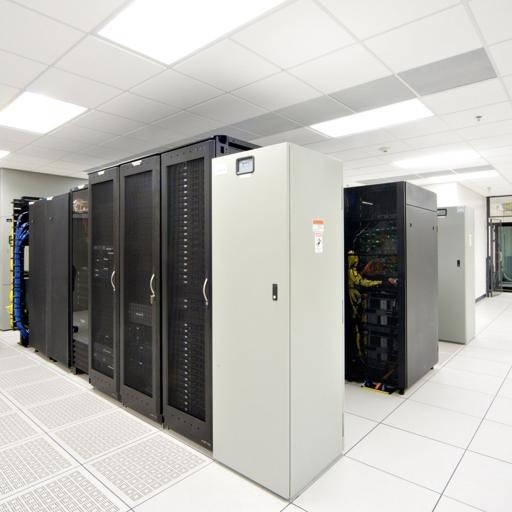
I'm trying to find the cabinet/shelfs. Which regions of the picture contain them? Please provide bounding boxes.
[207,142,344,502]
[157,133,213,457]
[431,204,475,344]
[28,192,71,371]
[67,187,90,381]
[341,178,439,395]
[85,152,163,428]
